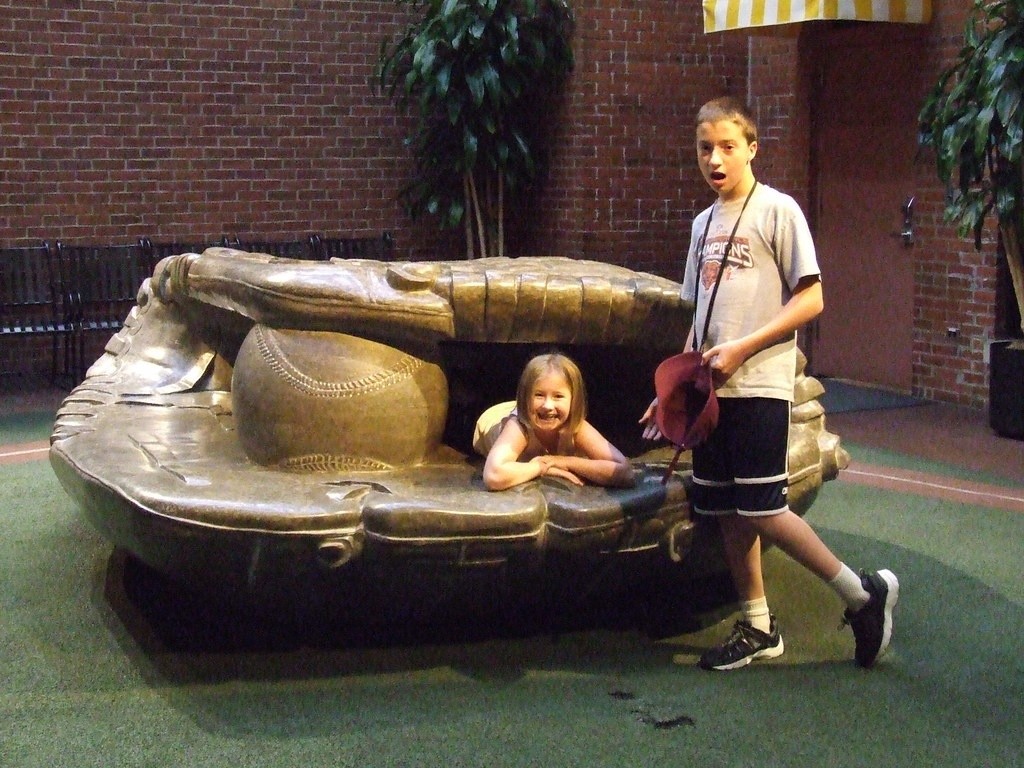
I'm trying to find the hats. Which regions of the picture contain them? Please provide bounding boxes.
[654,351,720,487]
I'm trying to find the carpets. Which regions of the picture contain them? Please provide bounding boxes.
[815,380,932,415]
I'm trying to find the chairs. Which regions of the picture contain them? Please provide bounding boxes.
[0,231,394,401]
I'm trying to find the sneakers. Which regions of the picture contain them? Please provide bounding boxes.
[841,567,900,669]
[699,613,785,671]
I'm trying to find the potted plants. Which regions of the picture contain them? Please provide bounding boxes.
[918,2,1024,438]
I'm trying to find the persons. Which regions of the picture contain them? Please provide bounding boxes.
[472,353,634,491]
[638,96,900,670]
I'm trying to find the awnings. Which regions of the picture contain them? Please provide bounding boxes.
[702,0,932,35]
